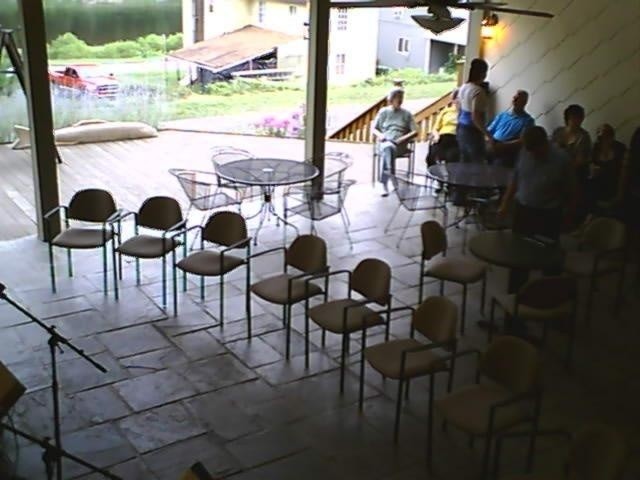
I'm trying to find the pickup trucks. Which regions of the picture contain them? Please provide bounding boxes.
[49,59,126,103]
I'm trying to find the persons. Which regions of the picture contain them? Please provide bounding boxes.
[492,126,579,333]
[367,85,418,197]
[450,60,640,235]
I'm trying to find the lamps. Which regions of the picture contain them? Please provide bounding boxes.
[480,10,496,39]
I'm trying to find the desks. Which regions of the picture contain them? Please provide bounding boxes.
[464,231,563,298]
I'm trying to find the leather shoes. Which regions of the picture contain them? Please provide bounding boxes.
[389,178,399,191]
[381,188,389,197]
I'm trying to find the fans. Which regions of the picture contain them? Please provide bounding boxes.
[329,0,555,36]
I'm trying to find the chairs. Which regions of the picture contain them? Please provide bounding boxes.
[170,211,253,328]
[417,219,488,333]
[109,195,188,306]
[305,257,393,393]
[246,234,330,361]
[559,216,633,311]
[492,416,629,480]
[376,127,639,230]
[168,144,357,251]
[358,295,459,444]
[426,335,545,480]
[43,189,122,298]
[473,273,589,361]
